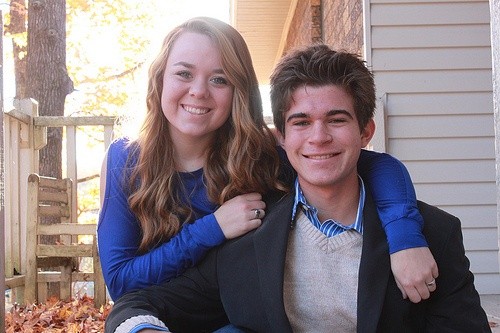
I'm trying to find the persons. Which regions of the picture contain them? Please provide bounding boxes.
[94,18,438,333]
[104,43,493,333]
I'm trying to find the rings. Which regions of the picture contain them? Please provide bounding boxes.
[254,208,260,219]
[427,278,436,288]
